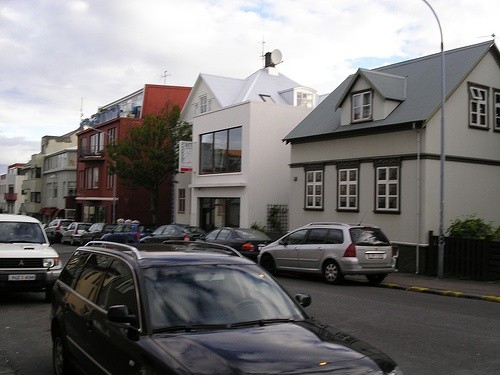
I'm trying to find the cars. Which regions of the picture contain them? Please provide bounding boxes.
[257,221,399,285]
[195,226,276,264]
[139,223,209,244]
[100,222,154,244]
[60,221,94,246]
[78,222,118,246]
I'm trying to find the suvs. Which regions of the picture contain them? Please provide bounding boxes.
[44,218,78,244]
[0,213,64,303]
[50,240,403,375]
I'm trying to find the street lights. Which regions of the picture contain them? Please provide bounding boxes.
[83,124,113,139]
